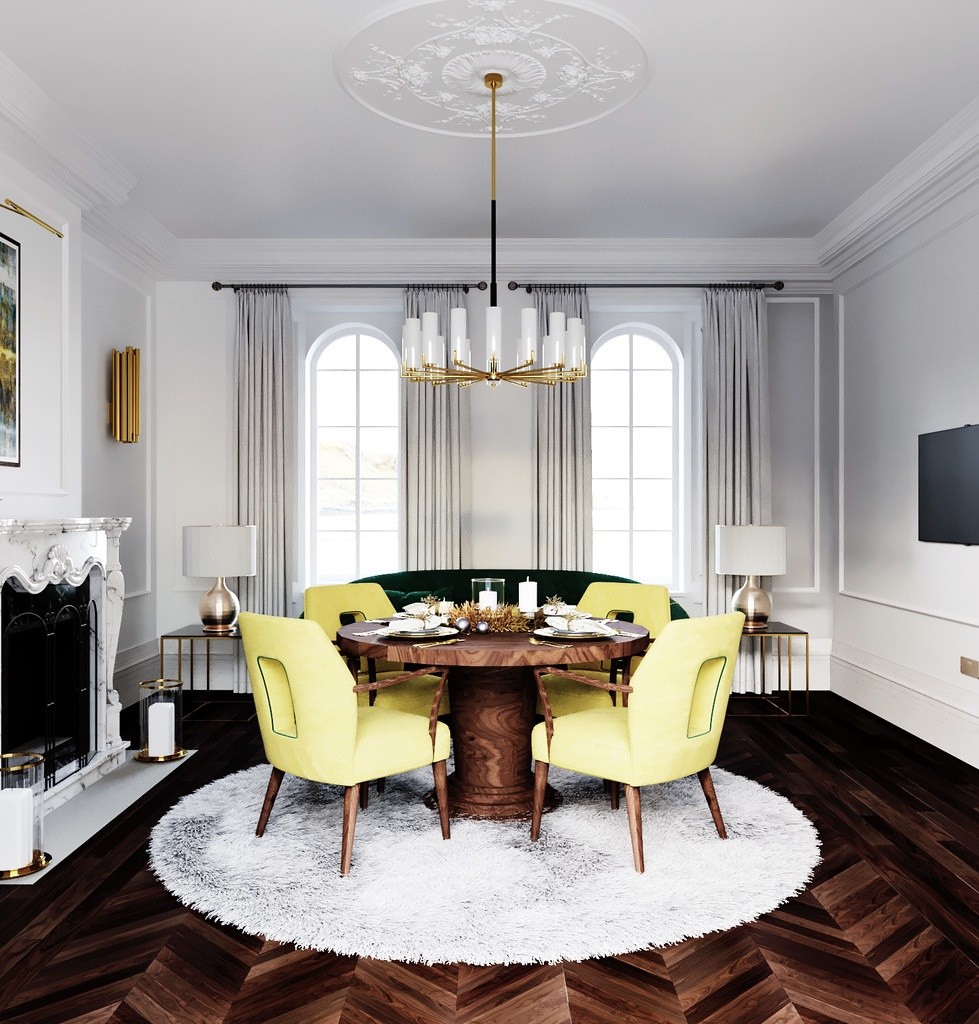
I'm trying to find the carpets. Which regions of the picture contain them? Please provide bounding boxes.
[147,736,824,966]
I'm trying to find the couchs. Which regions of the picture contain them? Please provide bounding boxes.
[300,569,689,723]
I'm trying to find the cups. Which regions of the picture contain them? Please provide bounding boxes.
[470,578,505,611]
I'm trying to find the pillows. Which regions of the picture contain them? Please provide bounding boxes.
[386,586,454,612]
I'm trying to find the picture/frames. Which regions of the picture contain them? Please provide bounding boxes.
[0,232,22,466]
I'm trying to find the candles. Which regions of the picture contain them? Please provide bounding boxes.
[479,586,498,612]
[0,781,34,869]
[149,696,176,757]
[518,577,538,612]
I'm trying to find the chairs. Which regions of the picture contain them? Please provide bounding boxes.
[528,609,746,873]
[305,585,449,793]
[537,582,669,794]
[235,611,451,878]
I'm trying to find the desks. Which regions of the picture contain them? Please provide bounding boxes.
[157,624,258,722]
[727,619,808,719]
[344,617,651,817]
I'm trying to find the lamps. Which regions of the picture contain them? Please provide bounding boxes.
[712,524,786,630]
[182,525,258,631]
[401,71,592,387]
[113,343,140,443]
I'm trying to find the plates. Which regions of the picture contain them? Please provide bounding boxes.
[375,627,460,638]
[533,627,617,638]
